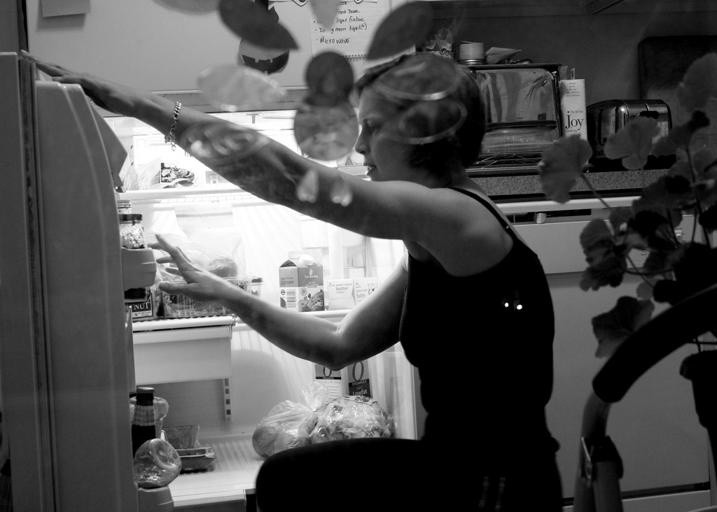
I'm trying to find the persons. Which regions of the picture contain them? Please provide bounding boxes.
[17,47,566,510]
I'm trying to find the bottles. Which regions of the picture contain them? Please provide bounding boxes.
[118,213,145,250]
[128,385,184,490]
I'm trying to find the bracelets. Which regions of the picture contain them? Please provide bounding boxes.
[164,100,182,145]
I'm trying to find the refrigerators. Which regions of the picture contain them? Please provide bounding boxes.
[0,51,421,511]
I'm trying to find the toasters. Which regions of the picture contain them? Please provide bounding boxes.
[586,99,674,171]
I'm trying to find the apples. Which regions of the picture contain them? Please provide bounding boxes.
[206,258,237,277]
